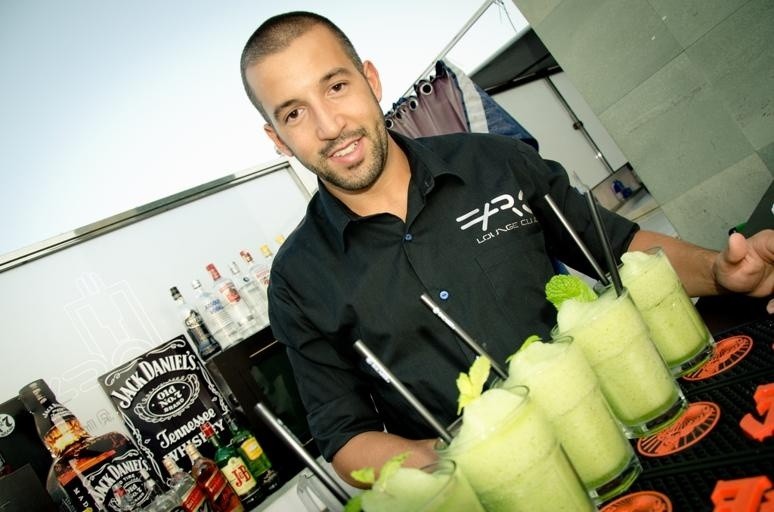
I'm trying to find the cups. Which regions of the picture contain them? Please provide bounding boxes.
[553,285,689,438]
[603,252,715,380]
[490,336,637,494]
[434,383,597,512]
[365,457,486,512]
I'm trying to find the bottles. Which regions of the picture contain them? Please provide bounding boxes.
[19,379,154,512]
[113,412,277,512]
[171,234,288,361]
[571,171,589,196]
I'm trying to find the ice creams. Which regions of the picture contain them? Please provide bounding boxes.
[611,251,709,366]
[547,275,677,421]
[501,335,626,486]
[345,454,485,512]
[443,356,594,512]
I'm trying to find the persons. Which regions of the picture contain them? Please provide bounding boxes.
[240,9,774,492]
